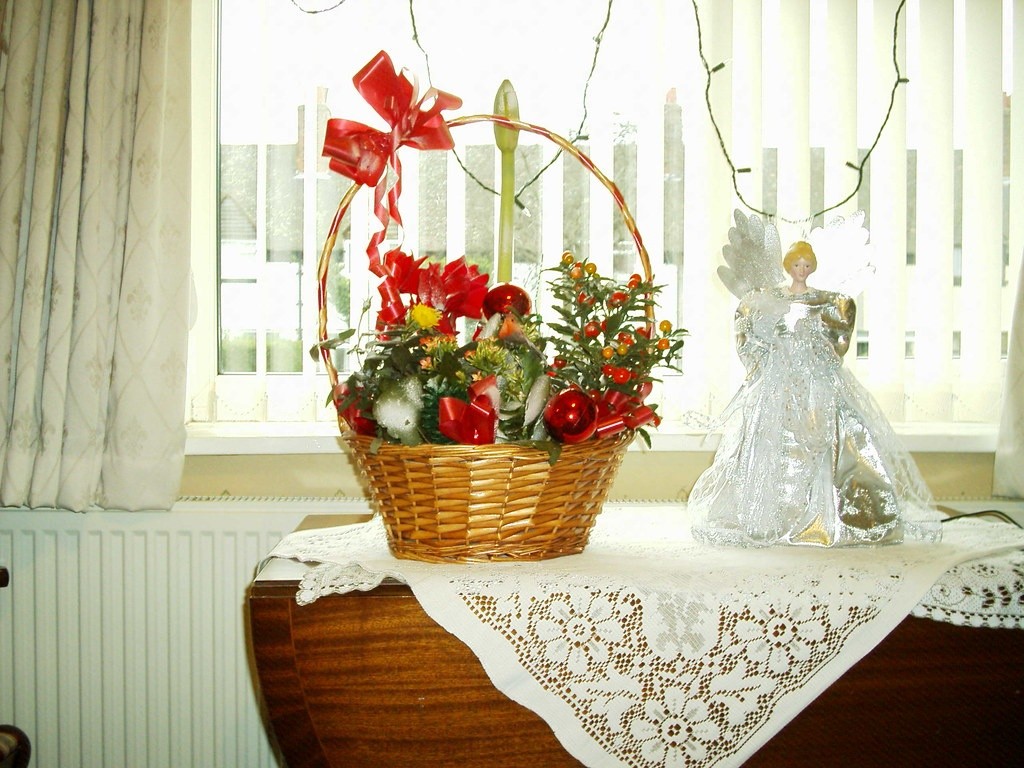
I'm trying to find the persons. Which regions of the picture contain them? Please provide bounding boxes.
[687,242,944,548]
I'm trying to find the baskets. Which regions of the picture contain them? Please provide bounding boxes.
[314,113,656,562]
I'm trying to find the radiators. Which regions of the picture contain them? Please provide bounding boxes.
[0,497,364,768]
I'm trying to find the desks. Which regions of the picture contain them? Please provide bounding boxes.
[243,510,1024,768]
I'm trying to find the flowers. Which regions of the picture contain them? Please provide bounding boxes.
[319,53,686,447]
